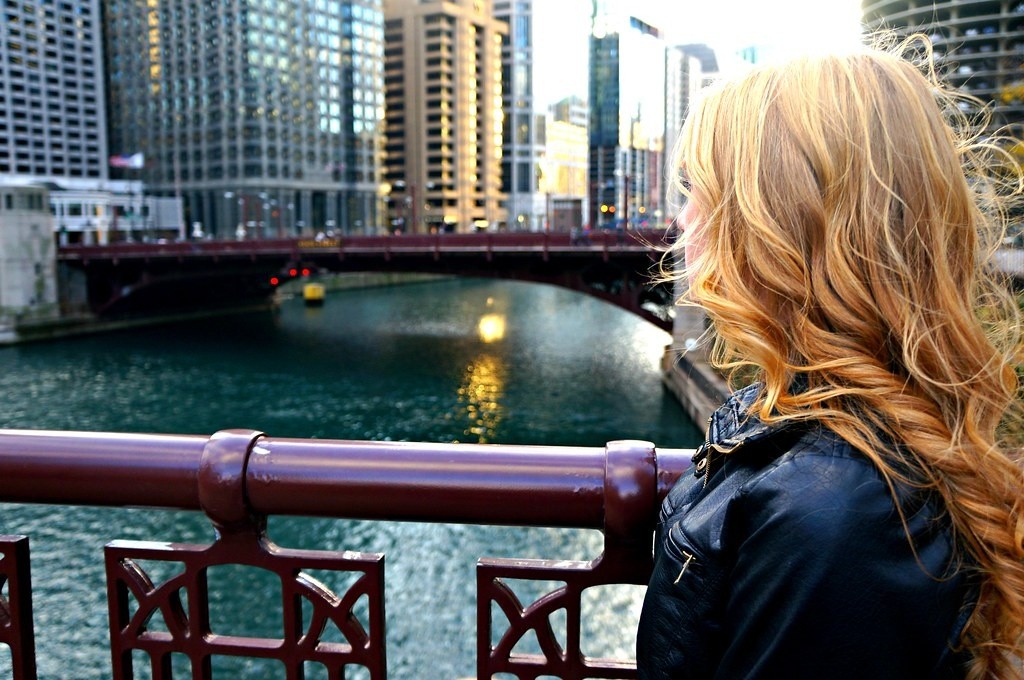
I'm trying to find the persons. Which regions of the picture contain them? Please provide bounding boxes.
[634,40,1024,680]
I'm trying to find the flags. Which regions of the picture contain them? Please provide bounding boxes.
[110,152,144,169]
